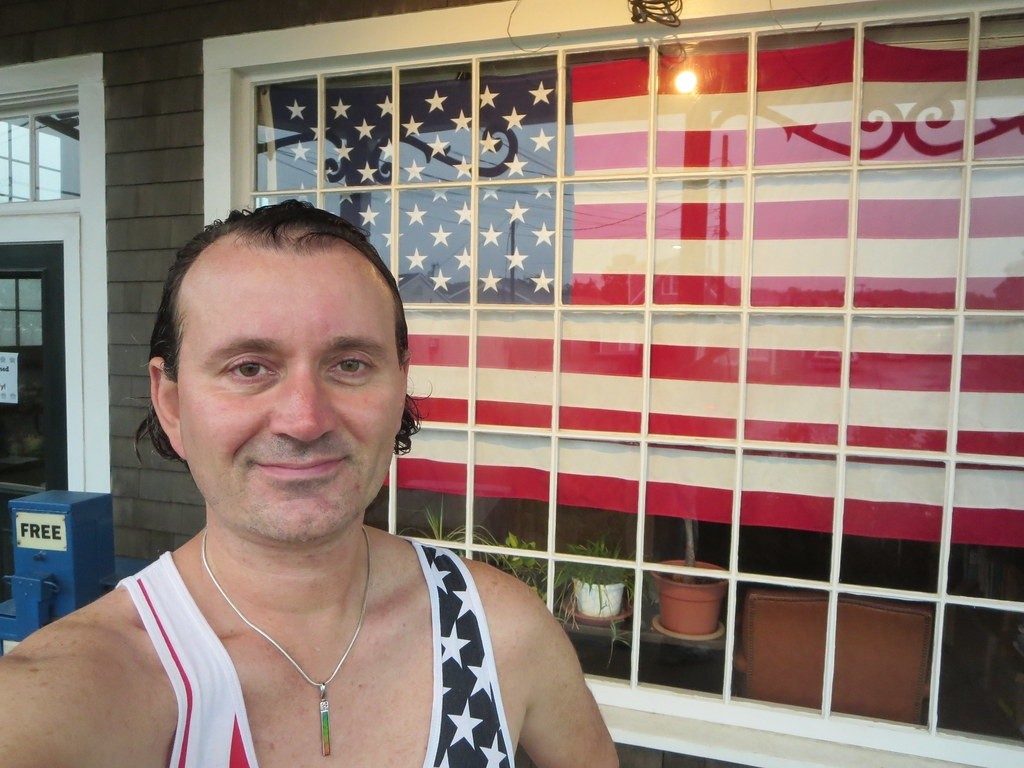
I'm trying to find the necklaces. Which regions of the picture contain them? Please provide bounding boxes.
[201,525,373,758]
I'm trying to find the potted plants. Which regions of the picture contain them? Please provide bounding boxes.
[648,516,726,643]
[540,537,634,627]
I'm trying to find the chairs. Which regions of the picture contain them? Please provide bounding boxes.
[739,587,932,724]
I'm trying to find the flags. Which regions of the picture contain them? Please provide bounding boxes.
[260,26,1023,547]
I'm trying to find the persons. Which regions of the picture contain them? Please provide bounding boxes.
[1,197,622,768]
